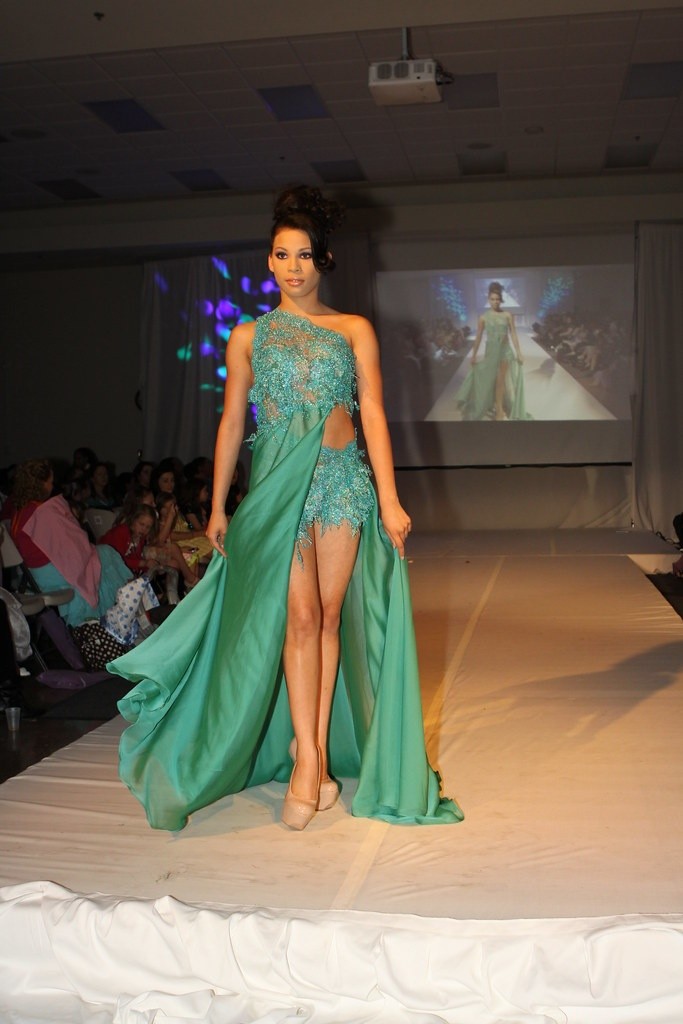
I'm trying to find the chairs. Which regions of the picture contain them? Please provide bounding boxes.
[81,508,120,540]
[0,525,74,677]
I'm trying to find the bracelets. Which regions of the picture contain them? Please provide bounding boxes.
[145,560,148,567]
[191,532,193,537]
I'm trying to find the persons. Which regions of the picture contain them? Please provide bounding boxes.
[454,283,533,420]
[105,178,464,833]
[534,309,635,400]
[381,318,472,417]
[0,444,248,646]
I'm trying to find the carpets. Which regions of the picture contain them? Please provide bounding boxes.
[39,674,141,723]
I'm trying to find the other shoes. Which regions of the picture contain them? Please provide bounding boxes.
[184,580,200,592]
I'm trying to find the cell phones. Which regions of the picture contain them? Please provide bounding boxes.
[188,547,199,553]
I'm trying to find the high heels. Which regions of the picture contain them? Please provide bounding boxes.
[279,745,322,831]
[288,737,339,811]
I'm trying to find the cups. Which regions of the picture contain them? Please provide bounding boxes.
[5,707,21,730]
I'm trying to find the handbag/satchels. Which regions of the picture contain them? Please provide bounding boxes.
[72,619,136,667]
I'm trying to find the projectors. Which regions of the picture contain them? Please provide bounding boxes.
[368,58,444,106]
